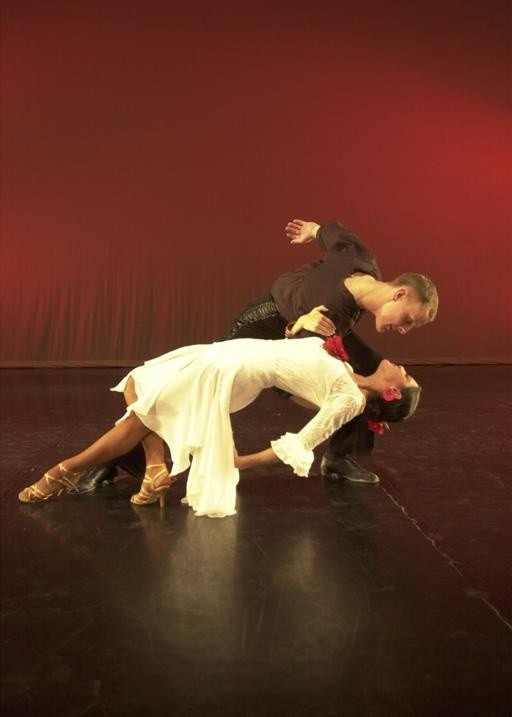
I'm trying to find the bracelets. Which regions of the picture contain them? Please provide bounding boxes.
[283,319,307,339]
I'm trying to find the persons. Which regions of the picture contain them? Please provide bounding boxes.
[17,304,424,518]
[67,217,441,500]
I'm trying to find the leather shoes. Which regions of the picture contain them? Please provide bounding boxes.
[320,454,380,484]
[67,463,119,495]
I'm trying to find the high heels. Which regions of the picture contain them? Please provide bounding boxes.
[130,463,172,509]
[19,463,81,502]
